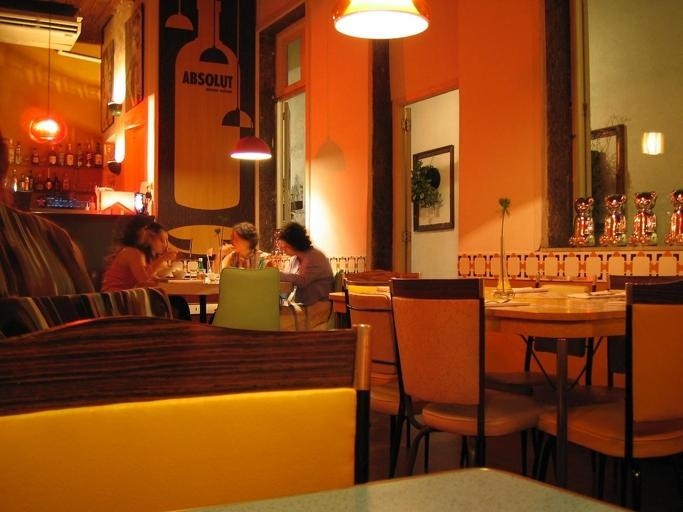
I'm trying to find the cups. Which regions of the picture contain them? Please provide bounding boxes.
[208,274,216,281]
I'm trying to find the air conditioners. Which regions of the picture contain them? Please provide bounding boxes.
[0,8,81,52]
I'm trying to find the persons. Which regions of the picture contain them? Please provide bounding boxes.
[211,221,272,274]
[105,223,178,292]
[98,215,153,288]
[279,222,336,332]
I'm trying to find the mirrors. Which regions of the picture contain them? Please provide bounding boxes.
[590,126,627,233]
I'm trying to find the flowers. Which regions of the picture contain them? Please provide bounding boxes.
[497,196,513,291]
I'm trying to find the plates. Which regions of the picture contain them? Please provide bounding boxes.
[166,277,189,280]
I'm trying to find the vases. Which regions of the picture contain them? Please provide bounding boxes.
[495,250,511,293]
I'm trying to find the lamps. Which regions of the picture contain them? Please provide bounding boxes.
[29,1,68,146]
[332,1,431,42]
[227,1,273,164]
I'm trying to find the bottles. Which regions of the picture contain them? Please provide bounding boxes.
[195,257,205,279]
[5,169,76,193]
[7,137,103,168]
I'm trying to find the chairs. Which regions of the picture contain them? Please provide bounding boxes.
[0,201,174,332]
[0,268,681,512]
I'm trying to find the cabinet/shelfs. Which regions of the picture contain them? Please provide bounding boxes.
[5,162,100,196]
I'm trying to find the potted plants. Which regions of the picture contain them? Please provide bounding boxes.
[410,162,443,207]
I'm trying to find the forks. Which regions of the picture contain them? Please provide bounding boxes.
[582,289,615,296]
[485,298,511,304]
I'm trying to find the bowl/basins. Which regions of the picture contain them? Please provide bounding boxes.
[171,272,186,279]
